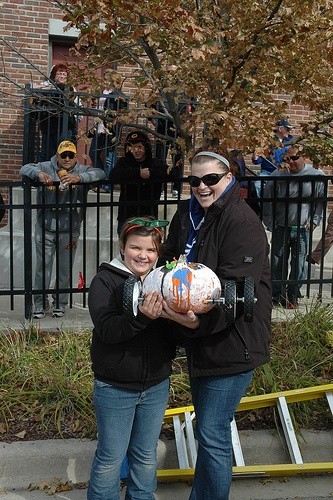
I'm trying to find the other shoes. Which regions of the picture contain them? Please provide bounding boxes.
[32,305,50,319]
[172,189,179,198]
[271,298,299,309]
[52,305,66,317]
[92,187,111,194]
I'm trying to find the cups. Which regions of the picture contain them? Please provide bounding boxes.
[55,168,70,188]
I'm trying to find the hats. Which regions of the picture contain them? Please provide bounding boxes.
[126,133,148,145]
[57,141,77,154]
[276,120,296,129]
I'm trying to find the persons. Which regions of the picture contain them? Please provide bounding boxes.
[262,147,325,309]
[157,146,270,500]
[251,120,294,173]
[109,130,167,238]
[19,140,106,319]
[307,210,333,265]
[87,214,177,500]
[228,149,260,217]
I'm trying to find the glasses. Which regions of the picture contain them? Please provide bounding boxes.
[187,172,228,188]
[287,154,302,161]
[60,153,75,159]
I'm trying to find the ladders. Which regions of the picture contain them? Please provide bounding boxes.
[156,383,333,482]
